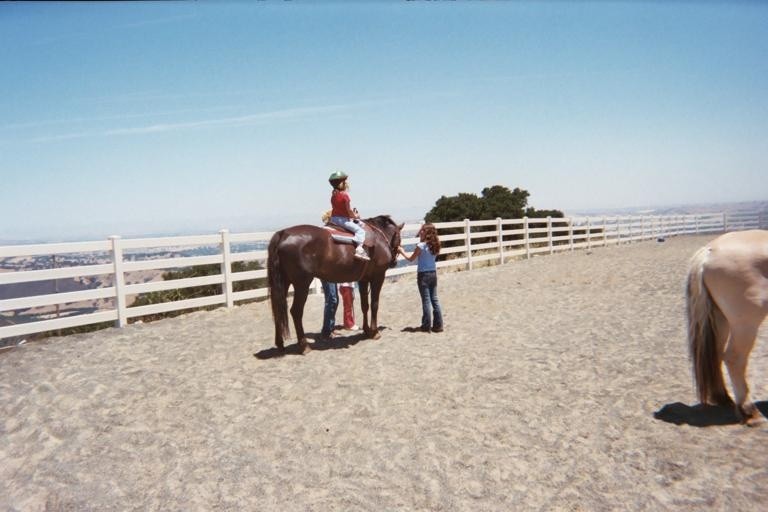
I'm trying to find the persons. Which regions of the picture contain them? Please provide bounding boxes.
[396,223,444,333]
[321,281,358,339]
[328,171,371,261]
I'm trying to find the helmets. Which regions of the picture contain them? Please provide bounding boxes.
[329,171,348,181]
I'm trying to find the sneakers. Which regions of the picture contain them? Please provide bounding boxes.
[321,330,341,339]
[344,325,359,330]
[355,251,371,261]
[418,327,444,332]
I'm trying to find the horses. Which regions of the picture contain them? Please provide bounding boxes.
[683,229,768,430]
[267,215,405,355]
